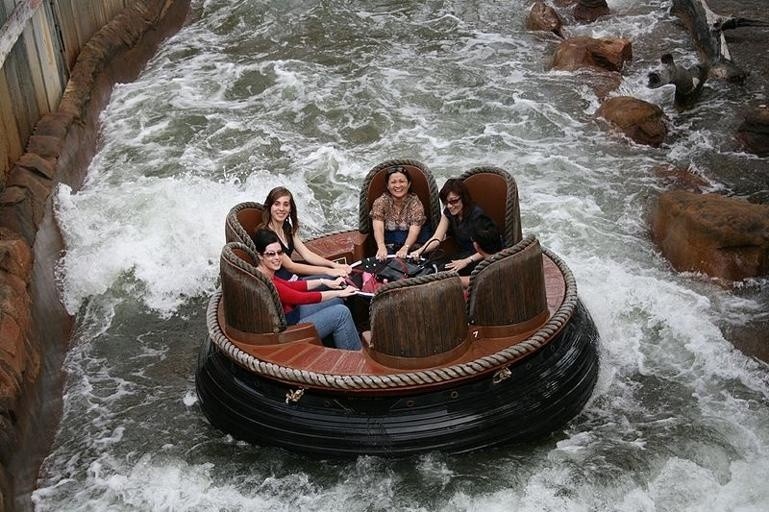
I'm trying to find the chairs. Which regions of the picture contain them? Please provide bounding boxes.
[354,159,441,259]
[362,271,469,369]
[468,234,549,337]
[446,166,521,248]
[225,202,265,255]
[220,242,318,345]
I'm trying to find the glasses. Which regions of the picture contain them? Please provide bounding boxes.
[386,167,406,173]
[444,197,460,205]
[263,250,283,256]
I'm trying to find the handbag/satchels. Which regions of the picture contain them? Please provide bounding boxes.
[379,238,445,281]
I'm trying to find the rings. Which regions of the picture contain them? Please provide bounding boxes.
[346,286,351,289]
[397,252,399,254]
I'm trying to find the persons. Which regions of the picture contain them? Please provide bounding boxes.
[457,215,504,304]
[254,228,364,352]
[252,186,353,290]
[367,165,431,262]
[408,179,504,277]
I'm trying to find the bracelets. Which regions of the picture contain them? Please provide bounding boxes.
[319,278,324,287]
[469,255,475,262]
[404,244,410,248]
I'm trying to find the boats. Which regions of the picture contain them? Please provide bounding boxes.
[197,158,600,469]
